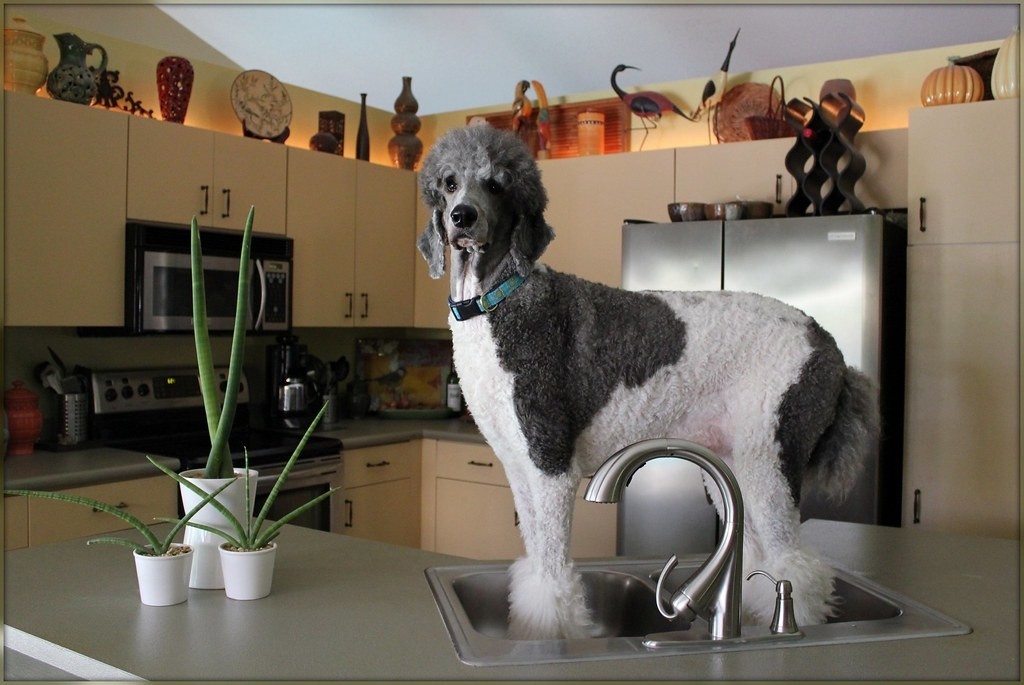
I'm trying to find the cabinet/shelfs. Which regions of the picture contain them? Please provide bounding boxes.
[417,145,674,325]
[5,472,179,552]
[4,88,129,327]
[674,126,907,211]
[127,114,287,245]
[287,146,415,330]
[421,436,618,562]
[341,439,420,547]
[904,98,1021,539]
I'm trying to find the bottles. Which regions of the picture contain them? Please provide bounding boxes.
[445,356,464,417]
[156,56,194,124]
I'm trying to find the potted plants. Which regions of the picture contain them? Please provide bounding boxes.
[177,206,259,593]
[146,400,343,602]
[4,478,239,606]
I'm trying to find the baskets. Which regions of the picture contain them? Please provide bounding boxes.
[745,74,800,139]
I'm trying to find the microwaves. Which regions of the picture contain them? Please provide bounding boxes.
[125,221,293,337]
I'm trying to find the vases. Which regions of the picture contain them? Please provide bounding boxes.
[355,93,370,161]
[820,79,858,104]
[386,77,425,171]
[156,56,194,124]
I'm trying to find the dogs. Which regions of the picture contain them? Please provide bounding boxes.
[415,126,885,641]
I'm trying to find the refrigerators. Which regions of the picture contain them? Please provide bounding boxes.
[621,208,906,528]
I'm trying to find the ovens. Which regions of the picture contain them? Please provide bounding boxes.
[252,457,346,537]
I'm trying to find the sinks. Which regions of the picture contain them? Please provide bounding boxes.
[423,559,698,668]
[648,559,974,655]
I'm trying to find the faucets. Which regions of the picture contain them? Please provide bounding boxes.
[582,436,743,638]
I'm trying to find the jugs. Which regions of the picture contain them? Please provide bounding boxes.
[279,377,318,416]
[45,33,108,106]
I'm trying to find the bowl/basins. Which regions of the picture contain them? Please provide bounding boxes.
[667,201,773,221]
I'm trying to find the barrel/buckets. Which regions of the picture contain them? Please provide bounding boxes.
[576,109,604,156]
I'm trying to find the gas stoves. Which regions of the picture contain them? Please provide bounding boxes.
[70,363,343,469]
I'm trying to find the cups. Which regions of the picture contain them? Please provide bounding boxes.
[322,394,338,422]
